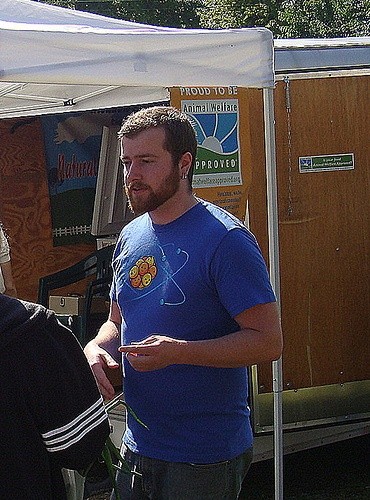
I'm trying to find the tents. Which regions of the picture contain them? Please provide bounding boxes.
[0,1,286,500]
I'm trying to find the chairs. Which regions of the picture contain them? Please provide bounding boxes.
[37,244,116,350]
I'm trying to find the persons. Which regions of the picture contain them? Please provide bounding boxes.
[0,292,117,500]
[84,107,282,500]
[0,225,18,298]
